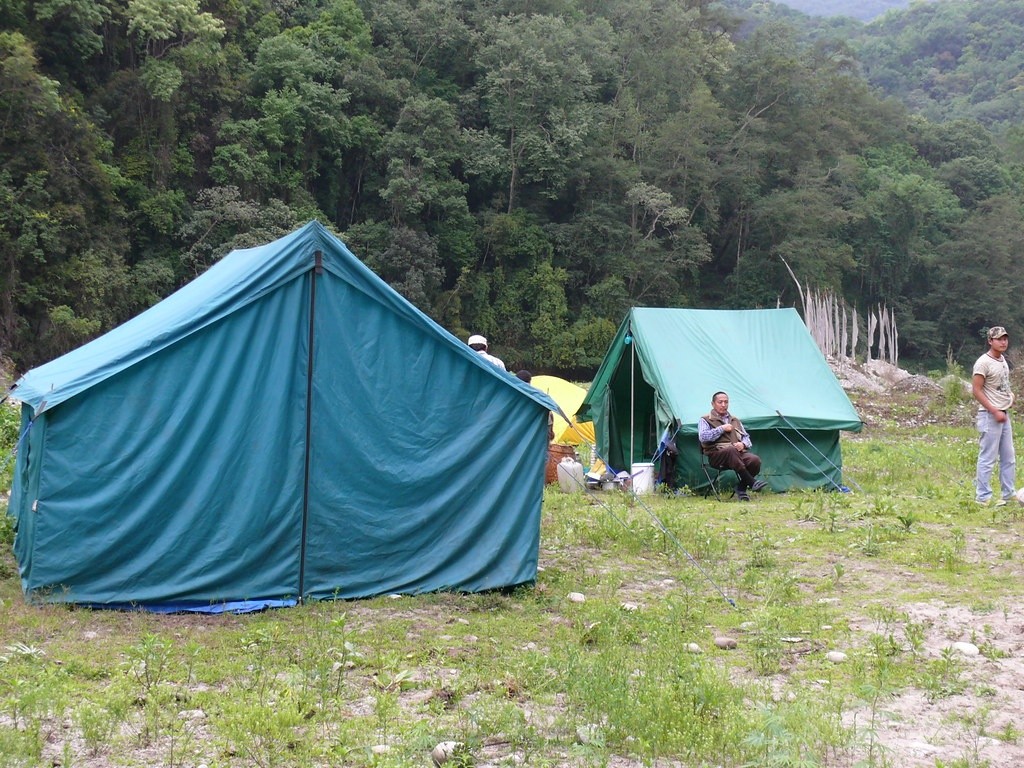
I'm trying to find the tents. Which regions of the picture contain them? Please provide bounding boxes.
[7,219,571,615]
[530,375,596,445]
[575,306,865,498]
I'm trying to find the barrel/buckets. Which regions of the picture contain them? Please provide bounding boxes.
[557,457,583,493]
[631,463,656,495]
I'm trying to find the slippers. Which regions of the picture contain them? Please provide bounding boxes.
[752,480,768,492]
[734,485,750,502]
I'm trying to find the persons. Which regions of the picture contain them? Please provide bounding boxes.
[467,335,506,372]
[516,370,531,385]
[698,391,770,501]
[972,326,1019,508]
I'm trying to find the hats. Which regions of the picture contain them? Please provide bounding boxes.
[468,335,488,352]
[517,370,531,382]
[987,326,1009,340]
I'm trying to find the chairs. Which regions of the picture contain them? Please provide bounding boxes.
[697,440,742,502]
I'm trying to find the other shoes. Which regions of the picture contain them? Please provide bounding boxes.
[975,497,1006,506]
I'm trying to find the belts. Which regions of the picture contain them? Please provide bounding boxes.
[977,409,1007,415]
